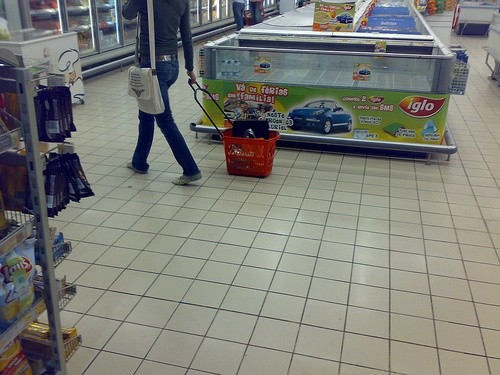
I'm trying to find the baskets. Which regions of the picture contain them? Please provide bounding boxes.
[188,78,281,178]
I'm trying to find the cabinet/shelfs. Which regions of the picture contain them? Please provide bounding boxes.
[0,53,84,375]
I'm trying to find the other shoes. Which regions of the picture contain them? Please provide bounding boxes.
[129,161,149,174]
[172,171,202,184]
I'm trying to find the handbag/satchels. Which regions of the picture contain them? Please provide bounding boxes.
[127,65,165,114]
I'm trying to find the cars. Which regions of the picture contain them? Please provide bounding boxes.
[285,99,352,135]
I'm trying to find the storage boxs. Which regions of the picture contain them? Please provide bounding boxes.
[372,6,410,15]
[414,0,457,16]
[366,16,417,29]
[0,321,78,375]
[448,58,471,96]
[357,26,422,35]
[312,1,357,32]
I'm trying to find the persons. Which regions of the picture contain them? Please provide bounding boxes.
[121,0,203,185]
[231,0,266,34]
